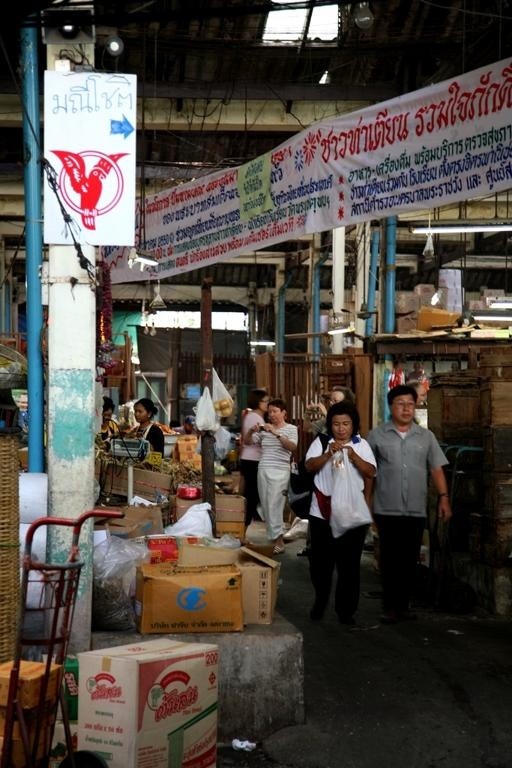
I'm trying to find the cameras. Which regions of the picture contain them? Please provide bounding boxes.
[258,426,267,431]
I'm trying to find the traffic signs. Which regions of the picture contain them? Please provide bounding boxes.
[44,70,138,246]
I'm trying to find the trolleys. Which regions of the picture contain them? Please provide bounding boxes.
[1,508,129,766]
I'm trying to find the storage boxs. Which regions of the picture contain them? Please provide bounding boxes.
[175,494,246,522]
[103,462,173,503]
[0,659,64,710]
[0,713,55,766]
[237,546,281,625]
[77,638,220,768]
[135,564,244,635]
[93,504,165,538]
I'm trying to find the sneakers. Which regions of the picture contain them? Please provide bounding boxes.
[334,613,359,629]
[376,602,415,627]
[296,546,309,558]
[270,545,287,556]
[308,601,327,623]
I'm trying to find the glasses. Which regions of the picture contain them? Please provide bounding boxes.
[391,400,416,409]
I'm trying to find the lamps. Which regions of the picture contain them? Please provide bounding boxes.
[430,288,443,306]
[149,280,168,309]
[327,327,355,336]
[319,71,330,84]
[260,9,310,48]
[474,315,512,321]
[305,4,342,47]
[408,225,512,234]
[250,341,276,347]
[106,36,124,56]
[354,1,375,29]
[127,248,159,272]
[485,297,512,309]
[143,310,157,336]
[60,23,77,39]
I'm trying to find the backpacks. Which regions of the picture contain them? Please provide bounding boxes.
[285,456,316,521]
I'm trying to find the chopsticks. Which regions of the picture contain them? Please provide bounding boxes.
[167,701,220,741]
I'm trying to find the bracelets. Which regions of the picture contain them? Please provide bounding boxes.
[277,434,283,438]
[439,493,448,497]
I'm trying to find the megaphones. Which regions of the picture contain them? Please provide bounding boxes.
[40,4,96,44]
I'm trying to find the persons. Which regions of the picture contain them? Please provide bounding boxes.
[101,397,118,434]
[238,390,269,525]
[172,415,195,433]
[366,384,453,623]
[245,399,299,553]
[306,401,376,622]
[298,386,353,555]
[127,398,164,460]
[390,363,429,405]
